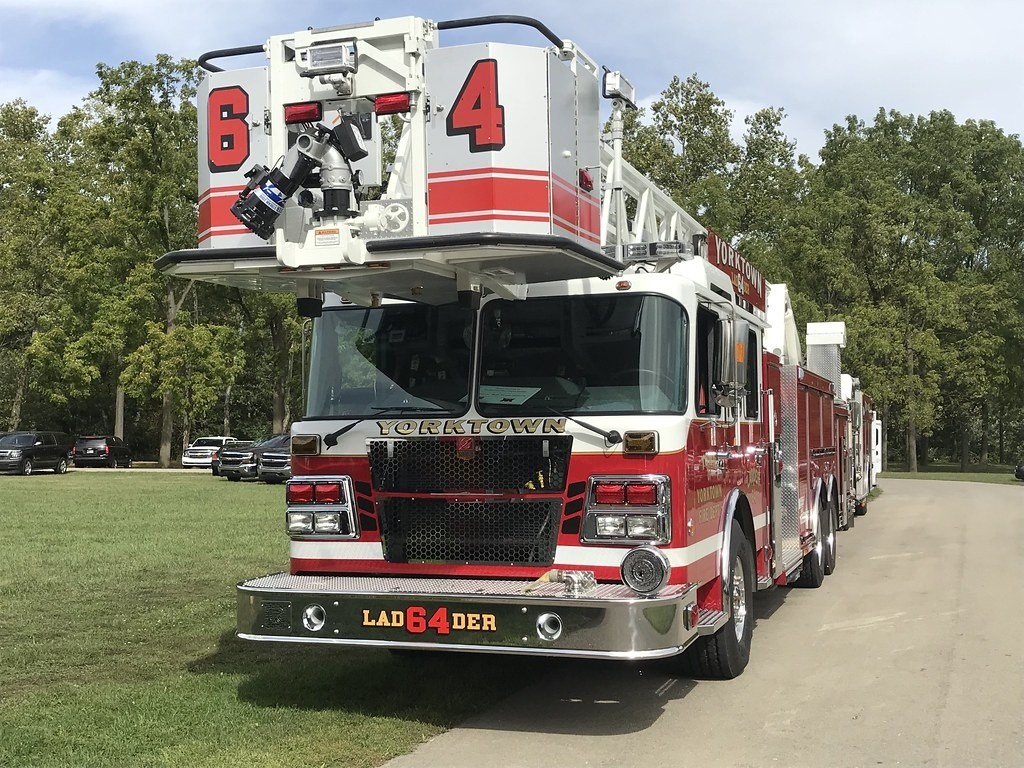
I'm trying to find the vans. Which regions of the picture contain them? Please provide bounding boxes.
[0,431,73,476]
[72,435,132,468]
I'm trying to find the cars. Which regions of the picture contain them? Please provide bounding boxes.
[181,436,238,469]
[212,444,249,476]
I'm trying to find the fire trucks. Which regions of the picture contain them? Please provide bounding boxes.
[158,12,882,682]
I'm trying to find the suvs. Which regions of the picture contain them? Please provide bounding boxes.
[217,434,287,481]
[256,436,292,484]
